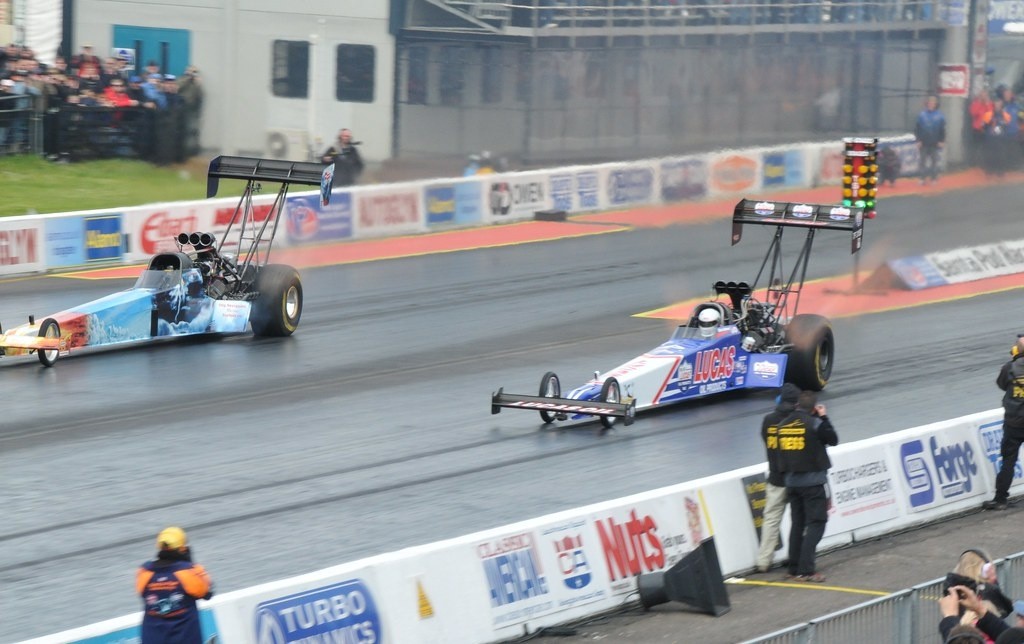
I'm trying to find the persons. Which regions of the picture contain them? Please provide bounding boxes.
[938,548,1024,644]
[180,270,208,312]
[877,82,1024,183]
[464,150,508,178]
[0,45,205,167]
[134,526,215,644]
[982,334,1024,509]
[694,308,721,342]
[321,129,363,187]
[753,383,839,582]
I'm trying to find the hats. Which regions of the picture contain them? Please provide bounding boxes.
[781,383,802,404]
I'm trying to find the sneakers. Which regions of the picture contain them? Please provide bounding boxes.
[983,498,1008,511]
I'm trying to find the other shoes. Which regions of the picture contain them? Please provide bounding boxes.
[793,571,828,583]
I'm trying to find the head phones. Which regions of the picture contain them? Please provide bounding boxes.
[960,548,995,580]
[775,395,782,404]
[337,128,352,145]
[1010,334,1024,356]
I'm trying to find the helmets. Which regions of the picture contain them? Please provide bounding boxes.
[158,527,186,552]
[698,308,721,337]
[193,257,215,276]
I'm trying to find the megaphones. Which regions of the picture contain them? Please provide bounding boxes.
[637,536,731,617]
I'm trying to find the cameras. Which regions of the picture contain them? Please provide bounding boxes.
[945,589,963,601]
